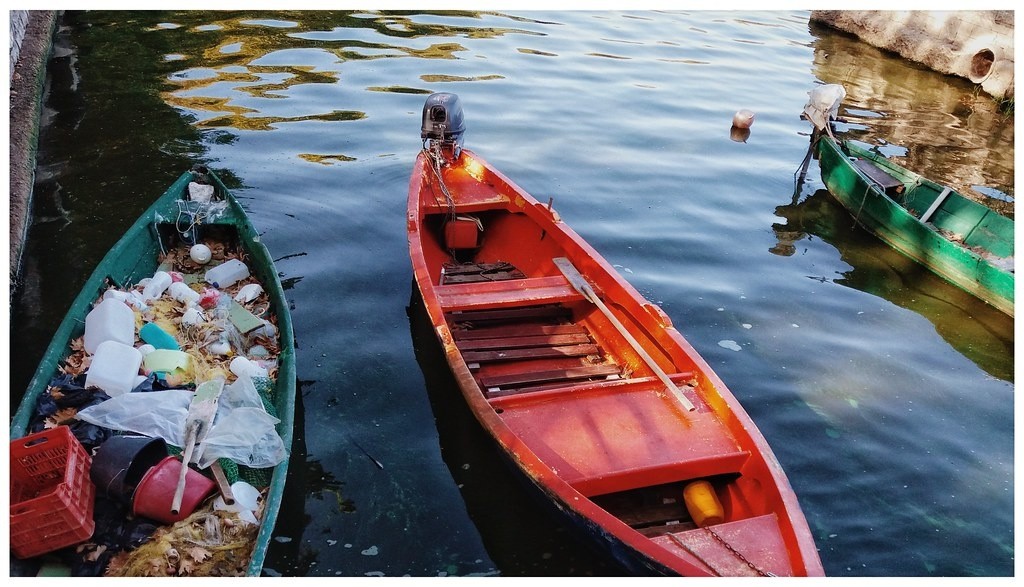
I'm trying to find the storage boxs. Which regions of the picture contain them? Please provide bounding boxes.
[10,425,95,559]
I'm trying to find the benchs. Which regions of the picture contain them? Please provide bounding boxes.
[487,370,751,498]
[434,276,604,313]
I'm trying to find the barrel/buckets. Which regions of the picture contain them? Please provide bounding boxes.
[204,258,251,288]
[131,455,215,524]
[214,481,262,516]
[88,435,168,505]
[85,341,143,397]
[84,297,136,354]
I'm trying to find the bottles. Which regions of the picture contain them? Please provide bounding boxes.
[143,271,173,300]
[211,342,233,357]
[139,322,182,351]
[139,344,156,361]
[230,357,271,393]
[127,295,148,312]
[103,291,134,303]
[167,281,201,304]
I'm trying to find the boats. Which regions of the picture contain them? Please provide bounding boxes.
[819,133,1014,318]
[406,139,826,577]
[10,162,298,577]
[790,187,1014,382]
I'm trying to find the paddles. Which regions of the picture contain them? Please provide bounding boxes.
[208,460,236,507]
[551,255,697,413]
[169,379,227,517]
